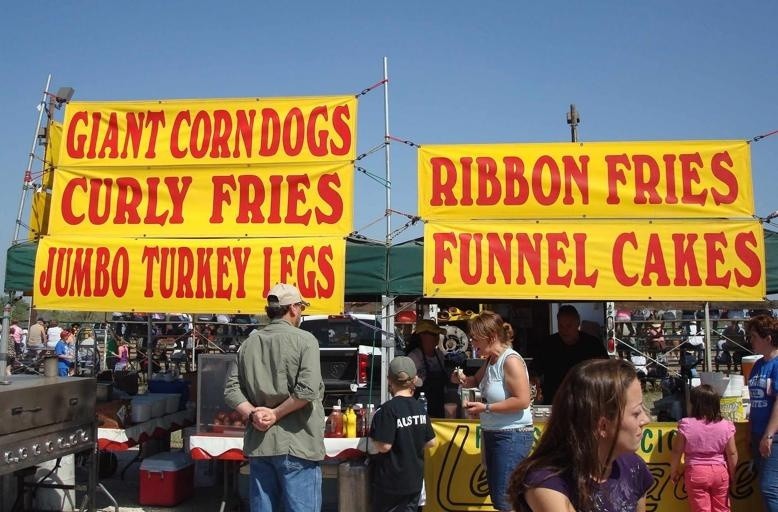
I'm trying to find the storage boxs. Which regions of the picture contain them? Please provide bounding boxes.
[146,379,189,410]
[138,449,195,506]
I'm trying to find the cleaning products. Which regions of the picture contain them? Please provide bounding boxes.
[343,405,357,437]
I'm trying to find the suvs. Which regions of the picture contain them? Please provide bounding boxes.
[107,310,194,351]
[292,312,407,414]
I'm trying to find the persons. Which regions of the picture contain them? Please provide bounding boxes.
[0,313,258,377]
[222,282,325,511]
[370,305,655,512]
[671,309,777,512]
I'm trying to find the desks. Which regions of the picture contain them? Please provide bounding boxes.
[96,403,196,482]
[190,432,368,512]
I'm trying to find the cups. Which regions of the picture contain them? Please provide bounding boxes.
[444,402,457,419]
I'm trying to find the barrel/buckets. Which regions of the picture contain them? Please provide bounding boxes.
[128,393,183,426]
[325,402,343,437]
[741,354,764,385]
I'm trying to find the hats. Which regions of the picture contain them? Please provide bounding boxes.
[60,331,70,337]
[387,355,423,388]
[412,319,447,335]
[265,282,308,309]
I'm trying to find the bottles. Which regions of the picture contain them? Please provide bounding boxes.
[418,391,428,412]
[329,402,376,438]
